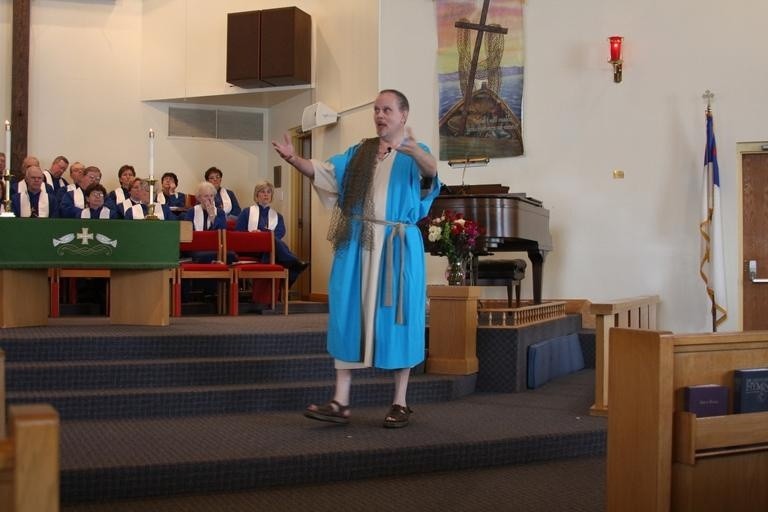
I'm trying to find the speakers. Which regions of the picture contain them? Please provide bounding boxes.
[260,6,312,87]
[226,10,275,89]
[301,101,340,133]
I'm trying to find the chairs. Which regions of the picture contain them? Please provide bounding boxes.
[169,190,291,318]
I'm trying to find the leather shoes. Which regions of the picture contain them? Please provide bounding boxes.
[300,260,310,271]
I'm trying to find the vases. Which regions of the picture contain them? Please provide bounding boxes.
[444,256,466,286]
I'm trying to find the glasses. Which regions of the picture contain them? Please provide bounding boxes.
[209,176,220,179]
[91,193,104,196]
[86,174,100,181]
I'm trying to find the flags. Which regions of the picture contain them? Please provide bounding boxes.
[697,109,728,331]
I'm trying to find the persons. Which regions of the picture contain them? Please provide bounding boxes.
[0,151,241,302]
[233,181,310,302]
[271,90,441,428]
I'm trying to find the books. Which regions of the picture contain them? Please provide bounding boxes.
[685,383,729,417]
[734,366,768,414]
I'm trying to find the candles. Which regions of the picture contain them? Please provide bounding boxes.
[149,129,154,176]
[4,119,11,171]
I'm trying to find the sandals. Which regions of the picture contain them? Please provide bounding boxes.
[304,401,352,423]
[383,404,413,427]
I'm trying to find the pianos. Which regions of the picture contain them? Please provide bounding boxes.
[418,187,551,304]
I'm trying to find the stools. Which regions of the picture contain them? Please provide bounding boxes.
[469,256,526,316]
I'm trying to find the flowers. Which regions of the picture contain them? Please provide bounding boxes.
[420,209,485,282]
